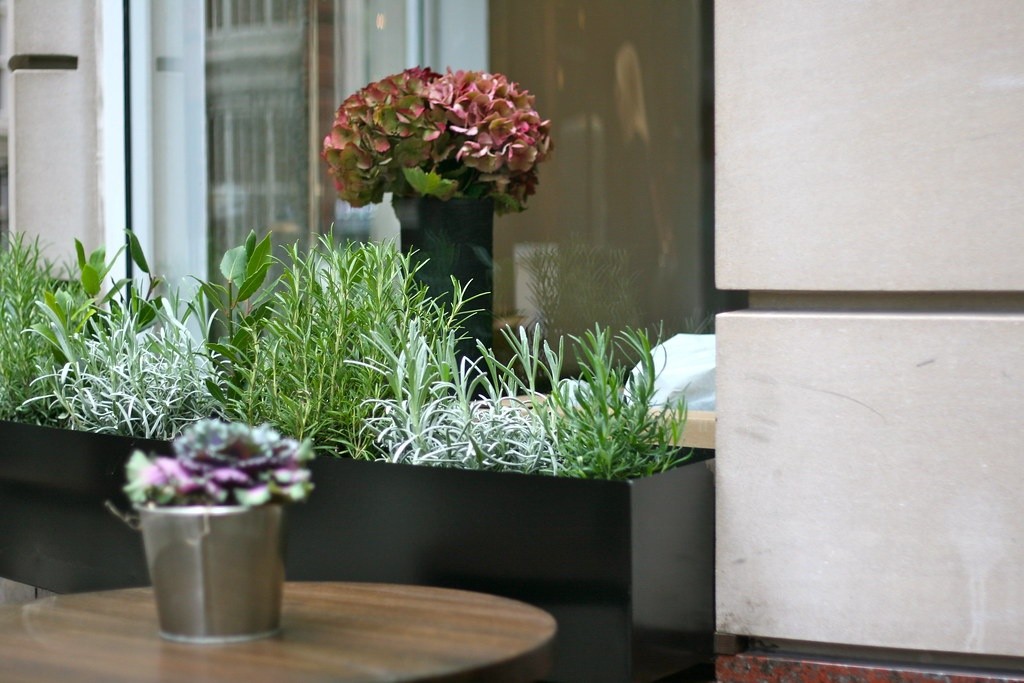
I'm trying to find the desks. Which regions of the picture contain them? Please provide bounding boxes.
[1,575,556,681]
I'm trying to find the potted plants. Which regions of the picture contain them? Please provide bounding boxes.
[1,218,717,683]
[124,412,314,641]
[320,63,550,402]
